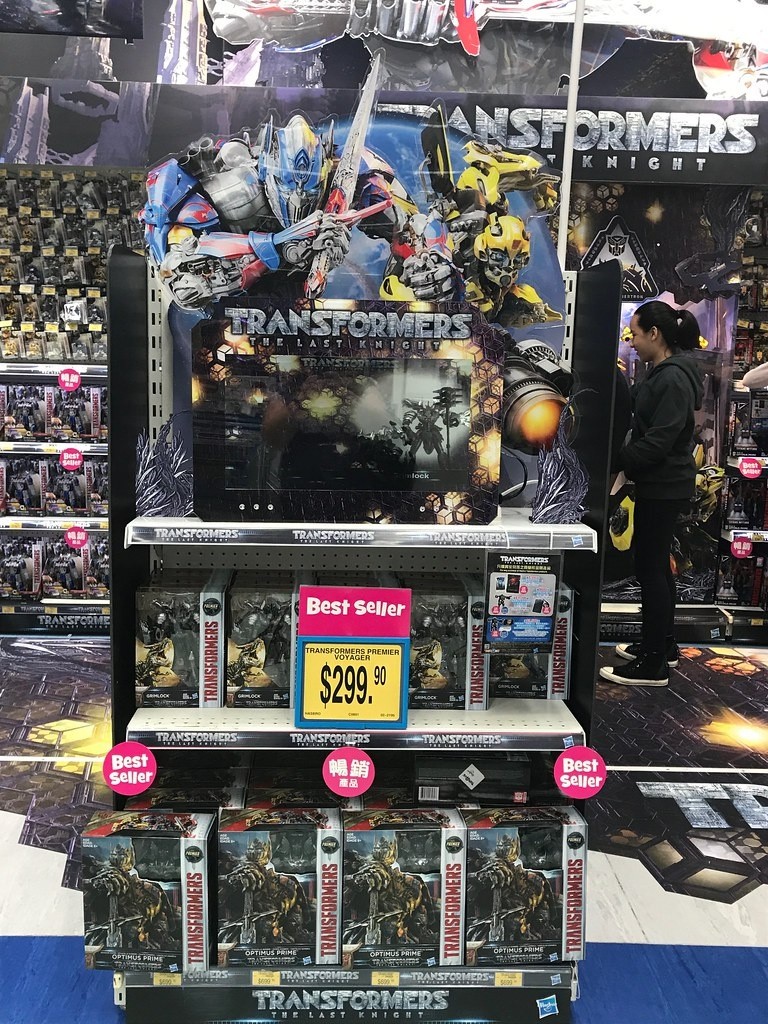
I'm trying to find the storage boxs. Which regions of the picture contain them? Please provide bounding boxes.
[716,379,768,607]
[81,751,588,974]
[134,561,575,710]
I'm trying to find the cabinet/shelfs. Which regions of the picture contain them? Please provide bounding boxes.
[714,260,768,647]
[0,359,111,637]
[107,245,624,1024]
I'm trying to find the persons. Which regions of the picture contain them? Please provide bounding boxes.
[599,300,703,688]
[743,361,768,392]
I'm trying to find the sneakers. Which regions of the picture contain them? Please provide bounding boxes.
[599,652,669,686]
[615,643,679,668]
